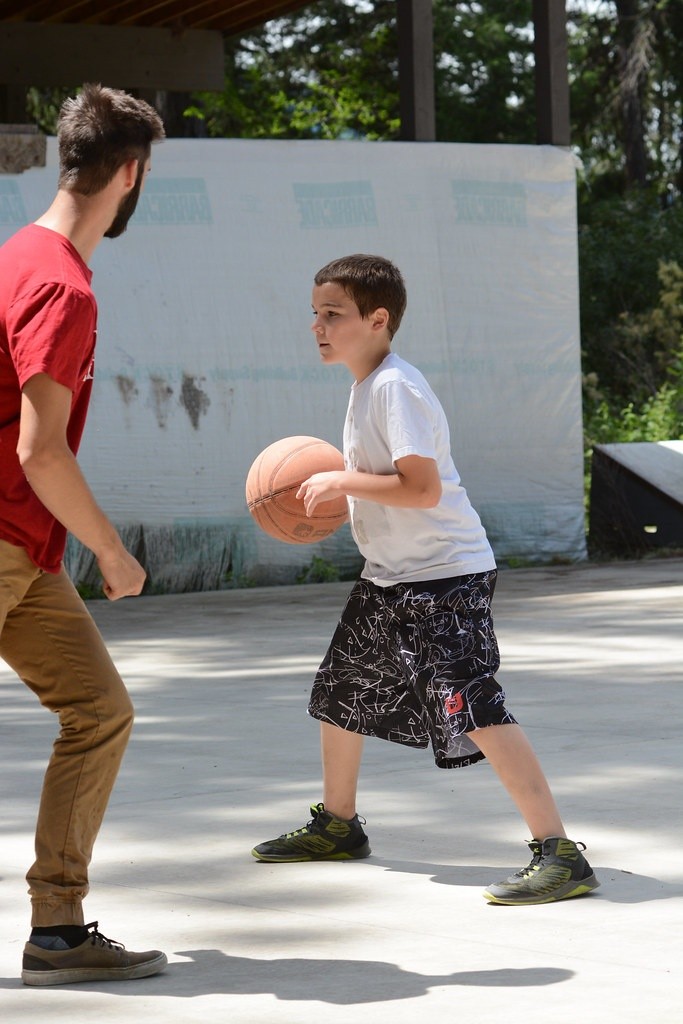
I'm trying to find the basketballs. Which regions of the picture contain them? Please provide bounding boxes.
[243,434,348,546]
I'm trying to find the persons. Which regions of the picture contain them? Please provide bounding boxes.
[253,254,602,906]
[0,89,167,987]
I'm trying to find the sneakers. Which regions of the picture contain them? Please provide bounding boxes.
[251,803,372,863]
[482,836,600,905]
[22,921,168,987]
